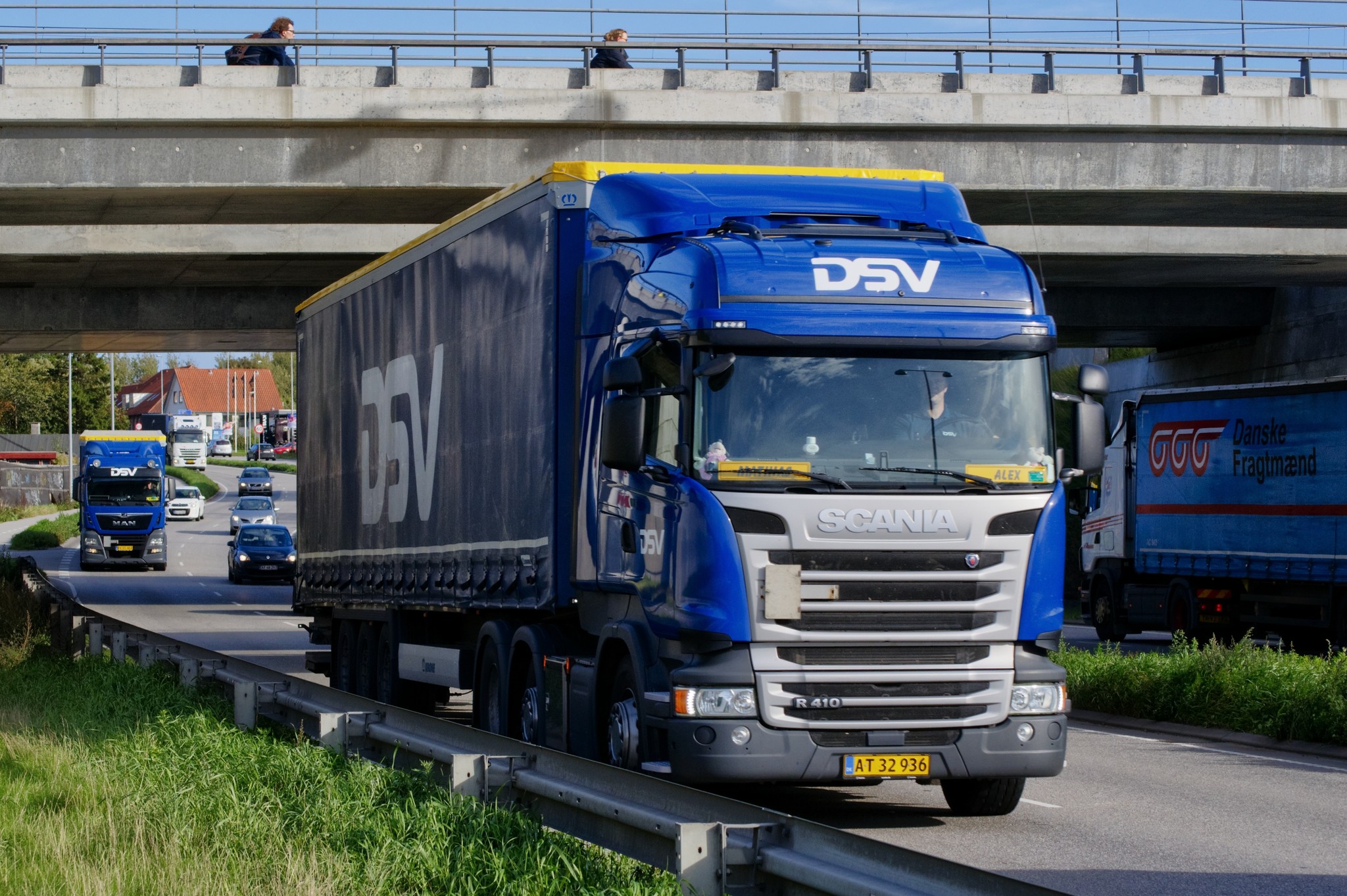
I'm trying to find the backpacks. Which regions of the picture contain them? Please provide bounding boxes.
[225,32,271,66]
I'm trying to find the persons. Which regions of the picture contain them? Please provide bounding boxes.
[590,28,634,68]
[141,480,159,496]
[890,367,995,450]
[227,16,295,66]
[258,500,271,510]
[188,491,194,498]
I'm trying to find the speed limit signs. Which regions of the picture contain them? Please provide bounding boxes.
[255,424,264,434]
[135,422,142,430]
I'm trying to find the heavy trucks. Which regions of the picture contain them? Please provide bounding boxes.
[290,160,1108,815]
[165,428,210,471]
[1078,373,1346,659]
[73,430,168,571]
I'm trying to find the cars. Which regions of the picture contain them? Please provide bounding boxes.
[163,486,205,522]
[246,443,277,461]
[274,442,296,454]
[228,496,282,536]
[236,467,275,496]
[207,439,232,457]
[226,523,298,586]
